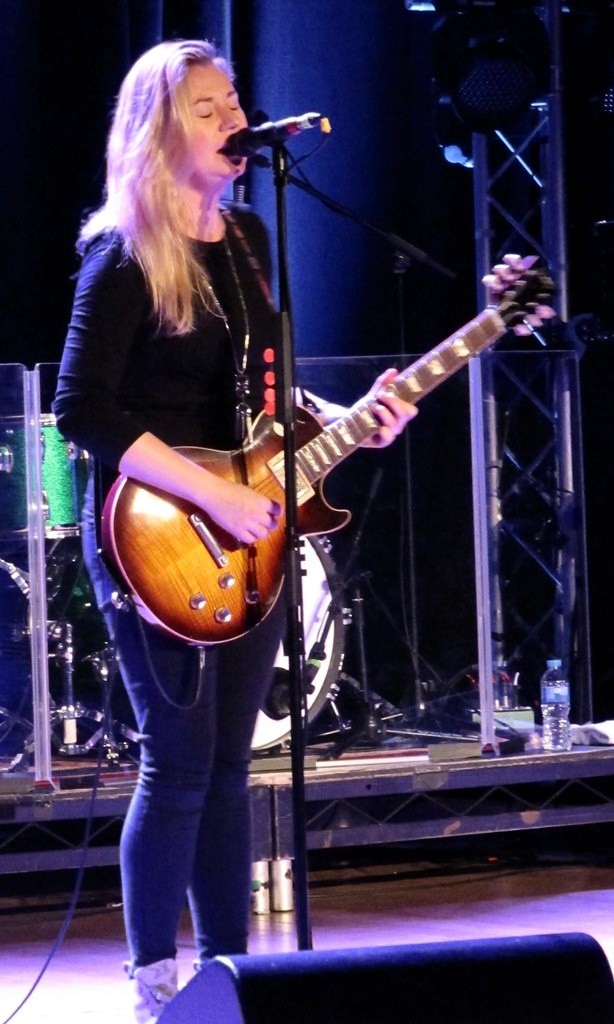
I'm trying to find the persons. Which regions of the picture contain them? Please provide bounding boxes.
[51,38,419,1022]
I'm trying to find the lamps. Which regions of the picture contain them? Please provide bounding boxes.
[440,144,473,167]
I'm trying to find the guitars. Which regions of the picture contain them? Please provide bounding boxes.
[99,249,562,646]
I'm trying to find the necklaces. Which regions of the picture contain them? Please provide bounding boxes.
[202,207,253,445]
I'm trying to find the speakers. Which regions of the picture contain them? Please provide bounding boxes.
[155,931,614,1024]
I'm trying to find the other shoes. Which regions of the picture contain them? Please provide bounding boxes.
[130,958,181,1024]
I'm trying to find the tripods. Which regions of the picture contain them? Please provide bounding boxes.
[5,624,141,776]
[301,465,480,762]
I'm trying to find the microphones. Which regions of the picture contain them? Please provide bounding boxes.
[228,112,323,157]
[273,686,295,714]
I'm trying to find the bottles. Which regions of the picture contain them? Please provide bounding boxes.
[540,660,574,751]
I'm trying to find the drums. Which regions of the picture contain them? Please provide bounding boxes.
[252,533,349,755]
[1,413,97,543]
